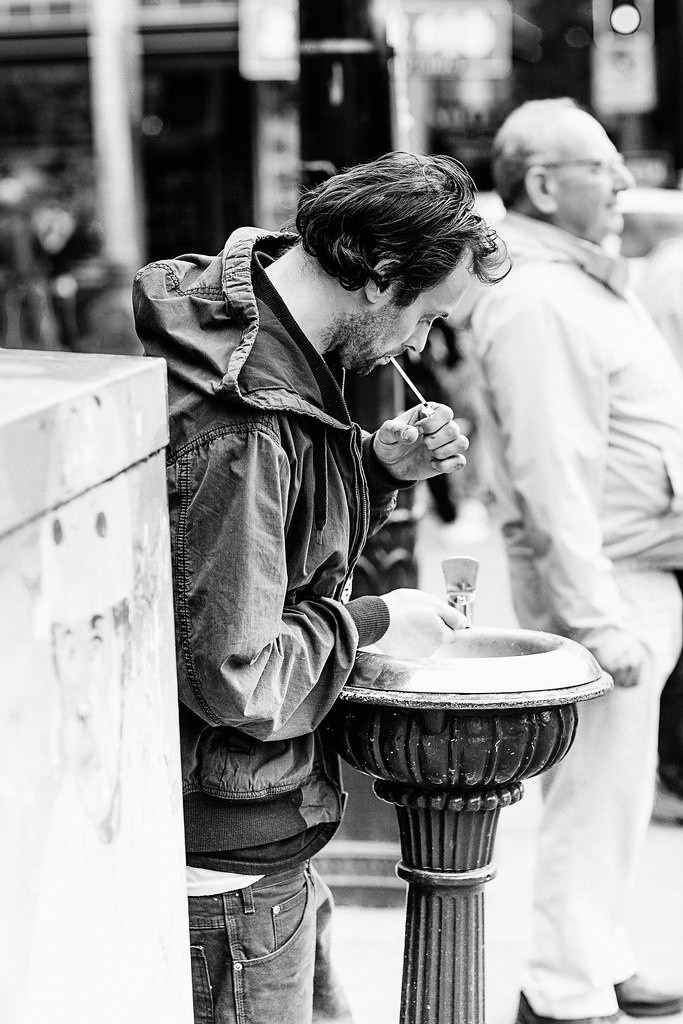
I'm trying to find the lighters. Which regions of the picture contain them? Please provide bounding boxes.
[420,407,435,417]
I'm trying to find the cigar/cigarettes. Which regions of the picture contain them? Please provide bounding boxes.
[390,356,427,408]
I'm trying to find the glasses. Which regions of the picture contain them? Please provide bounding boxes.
[523,154,628,178]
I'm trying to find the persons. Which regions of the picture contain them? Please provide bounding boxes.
[134,149,511,1024]
[449,97,683,1024]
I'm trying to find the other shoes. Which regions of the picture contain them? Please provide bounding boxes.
[651,782,683,823]
[612,976,682,1016]
[515,991,636,1024]
